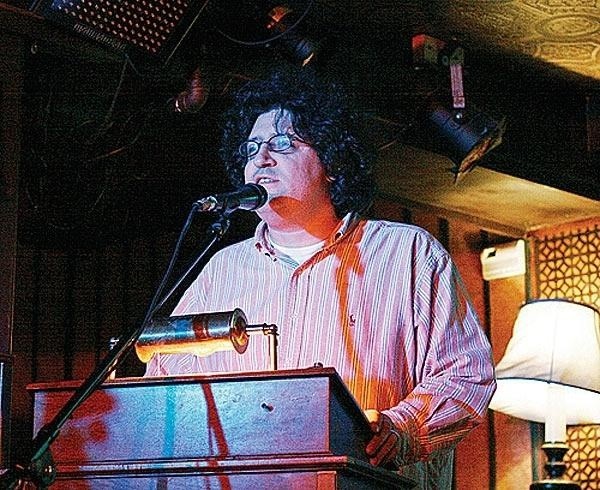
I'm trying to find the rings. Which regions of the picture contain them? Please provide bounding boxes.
[381,420,399,443]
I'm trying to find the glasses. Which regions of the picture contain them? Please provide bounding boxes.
[238,134,300,157]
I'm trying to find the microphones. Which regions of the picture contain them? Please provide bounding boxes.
[191,182,267,212]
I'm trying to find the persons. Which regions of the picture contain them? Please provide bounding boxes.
[143,75,498,475]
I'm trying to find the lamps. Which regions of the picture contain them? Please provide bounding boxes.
[484,298,600,489]
[402,86,509,185]
